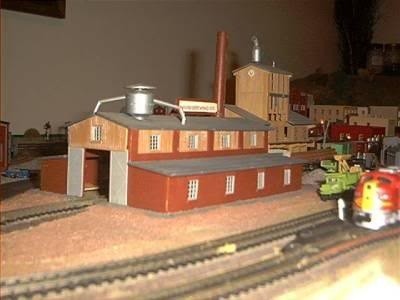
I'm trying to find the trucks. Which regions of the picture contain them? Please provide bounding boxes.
[319,172,359,200]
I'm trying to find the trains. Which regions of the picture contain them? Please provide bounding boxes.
[351,166,400,229]
